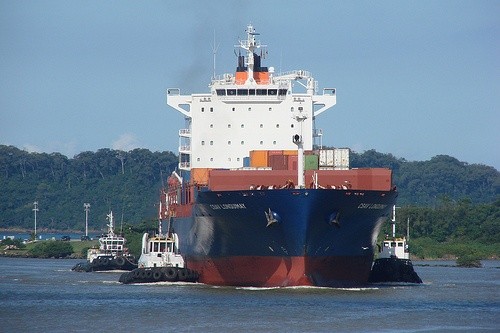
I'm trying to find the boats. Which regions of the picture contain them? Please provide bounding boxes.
[377,202,411,261]
[139,202,184,269]
[88,210,132,262]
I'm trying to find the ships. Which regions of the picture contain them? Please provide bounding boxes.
[160,24,393,287]
